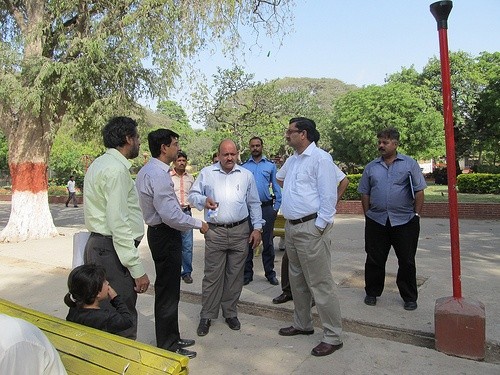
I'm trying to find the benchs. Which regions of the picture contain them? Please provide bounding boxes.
[254,215,287,257]
[0,297,190,375]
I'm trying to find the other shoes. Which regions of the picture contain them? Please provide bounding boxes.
[74,206,78,208]
[183,275,193,283]
[66,203,68,207]
[242,277,253,285]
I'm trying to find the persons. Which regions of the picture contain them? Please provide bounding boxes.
[168,151,195,284]
[64,265,133,334]
[278,117,343,357]
[187,138,266,337]
[84,116,150,343]
[212,151,219,164]
[66,175,79,209]
[357,130,427,310]
[273,128,349,304]
[0,312,67,375]
[240,137,283,287]
[136,128,209,360]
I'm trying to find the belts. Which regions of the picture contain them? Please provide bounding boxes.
[289,213,317,225]
[217,218,247,228]
[182,208,191,211]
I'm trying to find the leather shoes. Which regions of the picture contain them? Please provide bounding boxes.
[225,316,240,330]
[279,326,314,336]
[273,293,292,304]
[176,348,196,359]
[178,339,195,348]
[364,295,376,306]
[404,302,417,310]
[267,277,279,285]
[197,318,212,335]
[312,342,343,356]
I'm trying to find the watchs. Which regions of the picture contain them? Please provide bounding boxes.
[414,212,420,217]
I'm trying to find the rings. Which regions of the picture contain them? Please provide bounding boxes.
[140,288,144,291]
[257,242,260,245]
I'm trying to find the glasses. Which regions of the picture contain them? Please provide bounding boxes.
[287,129,302,135]
